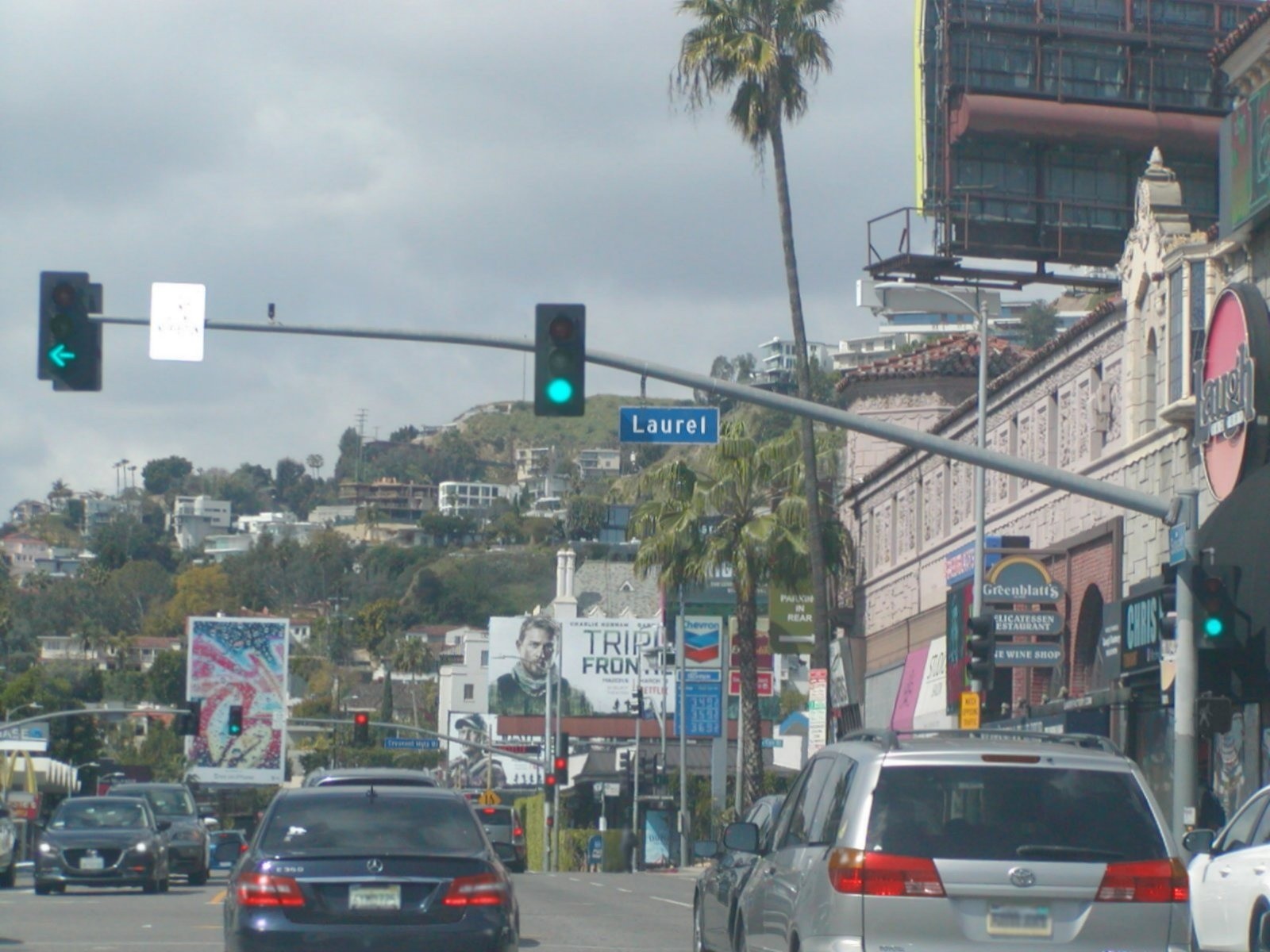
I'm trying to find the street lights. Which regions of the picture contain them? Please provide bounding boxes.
[4,702,44,759]
[871,281,980,738]
[490,650,563,872]
[67,759,102,798]
[95,772,126,796]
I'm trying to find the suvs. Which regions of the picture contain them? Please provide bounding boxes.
[103,781,215,887]
[722,724,1194,952]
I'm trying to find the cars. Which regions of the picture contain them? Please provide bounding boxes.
[202,765,530,877]
[32,795,173,895]
[1180,783,1270,952]
[0,799,20,889]
[692,792,790,952]
[221,781,521,952]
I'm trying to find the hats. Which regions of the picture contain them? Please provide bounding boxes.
[456,715,488,733]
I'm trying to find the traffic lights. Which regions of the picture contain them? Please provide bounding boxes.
[631,689,646,719]
[532,301,587,419]
[1195,695,1233,736]
[1157,560,1177,640]
[619,749,630,788]
[45,280,105,392]
[354,711,369,744]
[644,752,659,786]
[227,704,243,737]
[629,757,646,784]
[965,610,998,683]
[1190,563,1239,651]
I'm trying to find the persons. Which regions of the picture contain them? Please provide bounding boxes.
[489,616,592,715]
[449,714,507,787]
